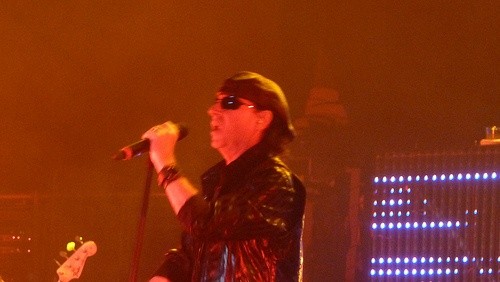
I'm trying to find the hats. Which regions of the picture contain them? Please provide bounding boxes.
[219,71,294,136]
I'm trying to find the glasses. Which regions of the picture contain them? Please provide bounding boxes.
[214,96,260,112]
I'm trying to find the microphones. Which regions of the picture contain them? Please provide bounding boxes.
[112,122,189,160]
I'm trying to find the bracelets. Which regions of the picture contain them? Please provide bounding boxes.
[157,163,183,190]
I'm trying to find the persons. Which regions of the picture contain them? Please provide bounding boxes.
[141,71,306,282]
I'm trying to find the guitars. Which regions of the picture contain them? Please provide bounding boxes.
[53,235,97,282]
